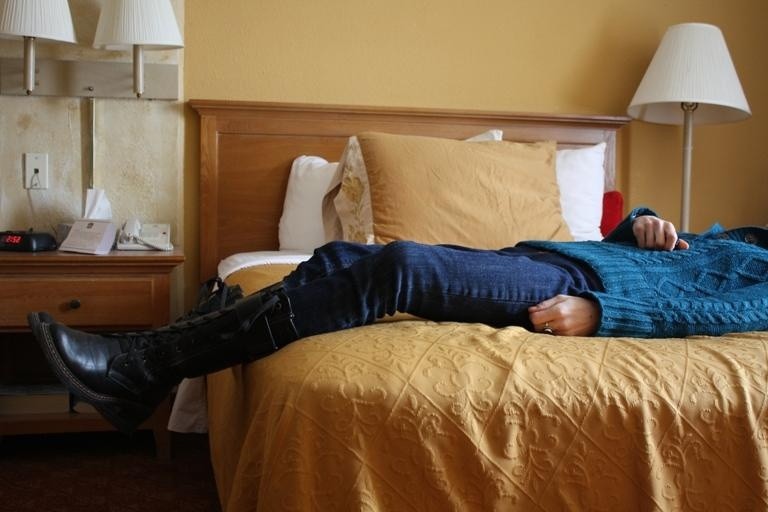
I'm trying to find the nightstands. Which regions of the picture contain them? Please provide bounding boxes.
[0,249,185,466]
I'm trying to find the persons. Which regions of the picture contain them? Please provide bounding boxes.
[27,206,767,445]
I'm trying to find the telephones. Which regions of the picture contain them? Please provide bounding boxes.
[117,219,173,251]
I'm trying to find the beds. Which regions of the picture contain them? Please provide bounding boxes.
[187,98,768,512]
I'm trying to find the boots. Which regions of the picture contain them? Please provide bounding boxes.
[24,275,244,342]
[34,283,306,436]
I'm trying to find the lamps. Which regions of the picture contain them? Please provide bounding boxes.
[627,21,752,235]
[1,0,184,98]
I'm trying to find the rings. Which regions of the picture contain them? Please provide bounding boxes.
[544,322,552,333]
